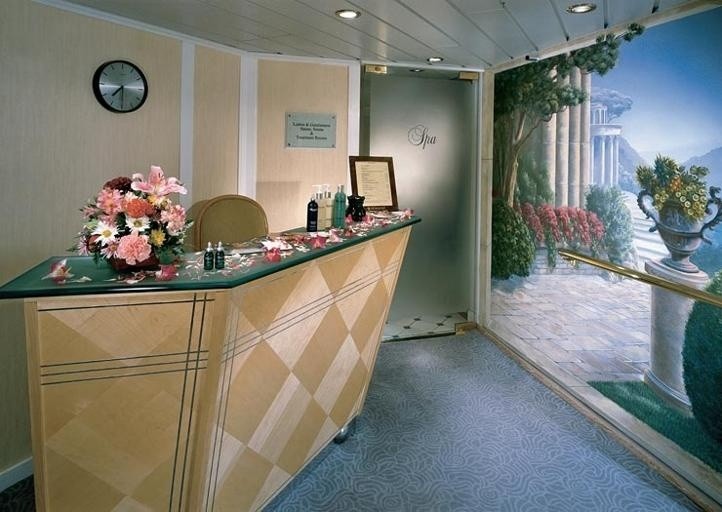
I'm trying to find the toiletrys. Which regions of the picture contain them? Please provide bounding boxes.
[204,239,224,271]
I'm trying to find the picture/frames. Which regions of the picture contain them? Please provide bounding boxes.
[349,156,399,213]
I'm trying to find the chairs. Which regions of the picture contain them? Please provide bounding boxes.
[195,194,269,253]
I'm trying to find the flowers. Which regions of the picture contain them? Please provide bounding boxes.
[521,201,604,248]
[64,163,195,265]
[637,154,722,223]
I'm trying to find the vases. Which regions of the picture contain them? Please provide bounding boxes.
[110,256,159,271]
[635,186,722,273]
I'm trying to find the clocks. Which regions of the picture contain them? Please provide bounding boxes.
[91,59,148,115]
